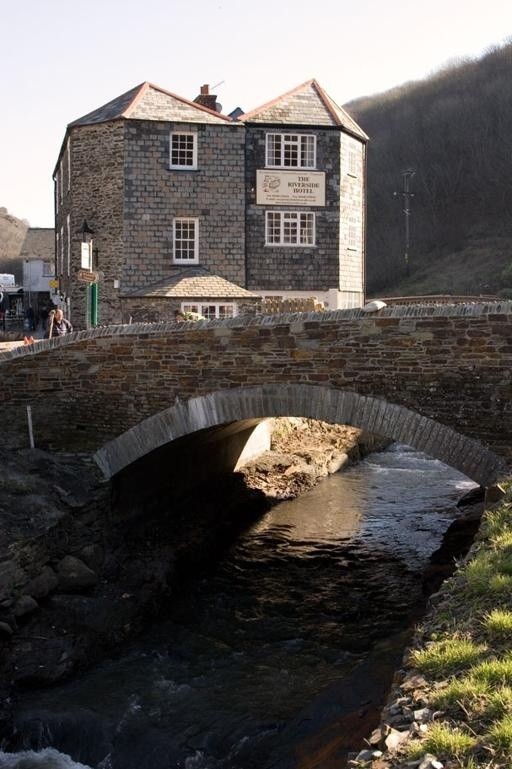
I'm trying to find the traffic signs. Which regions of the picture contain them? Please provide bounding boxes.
[77,270,100,286]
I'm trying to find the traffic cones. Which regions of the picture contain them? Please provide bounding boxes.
[23,336,36,345]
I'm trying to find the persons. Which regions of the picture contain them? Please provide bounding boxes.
[174,310,207,320]
[48,309,73,338]
[40,306,48,331]
[27,303,36,331]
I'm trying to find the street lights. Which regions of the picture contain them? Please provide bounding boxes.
[25,256,33,308]
